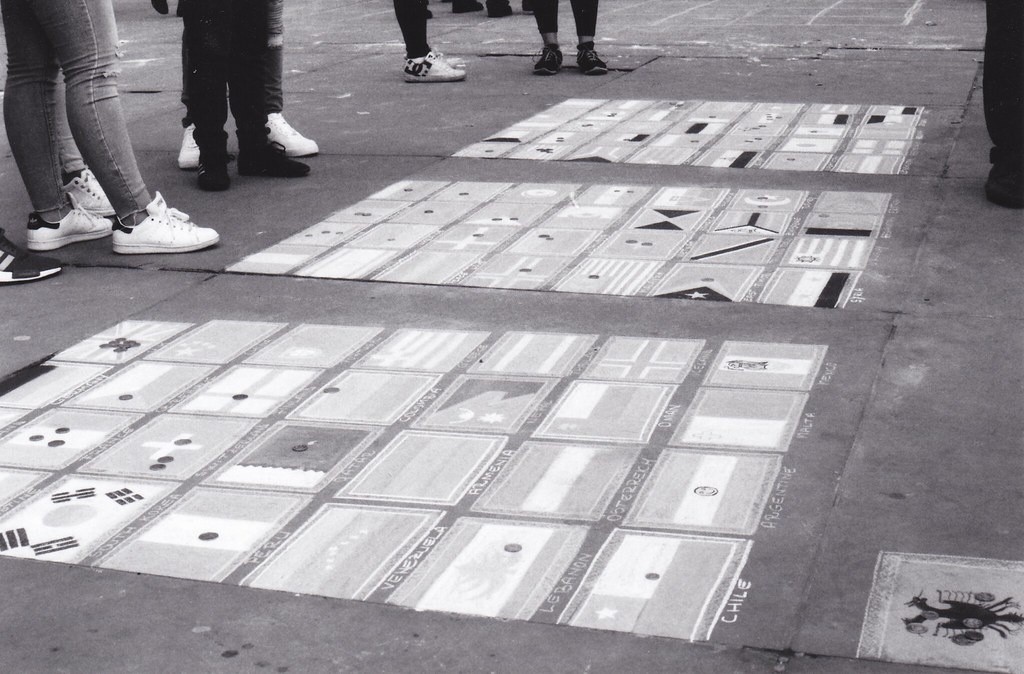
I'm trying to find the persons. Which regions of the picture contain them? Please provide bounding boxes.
[393,0,466,82]
[532,0,608,77]
[452,0,534,18]
[150,0,320,191]
[0,0,220,282]
[982,0,1024,208]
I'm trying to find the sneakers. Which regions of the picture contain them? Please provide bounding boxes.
[63,168,116,217]
[198,146,231,191]
[0,224,62,283]
[531,46,563,74]
[237,140,312,176]
[27,191,115,251]
[578,40,608,74]
[112,191,221,254]
[265,111,319,156]
[177,123,200,170]
[403,51,467,81]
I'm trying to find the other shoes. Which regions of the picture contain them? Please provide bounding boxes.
[451,3,484,14]
[426,10,432,18]
[488,6,514,18]
[522,4,534,15]
[985,161,1024,208]
